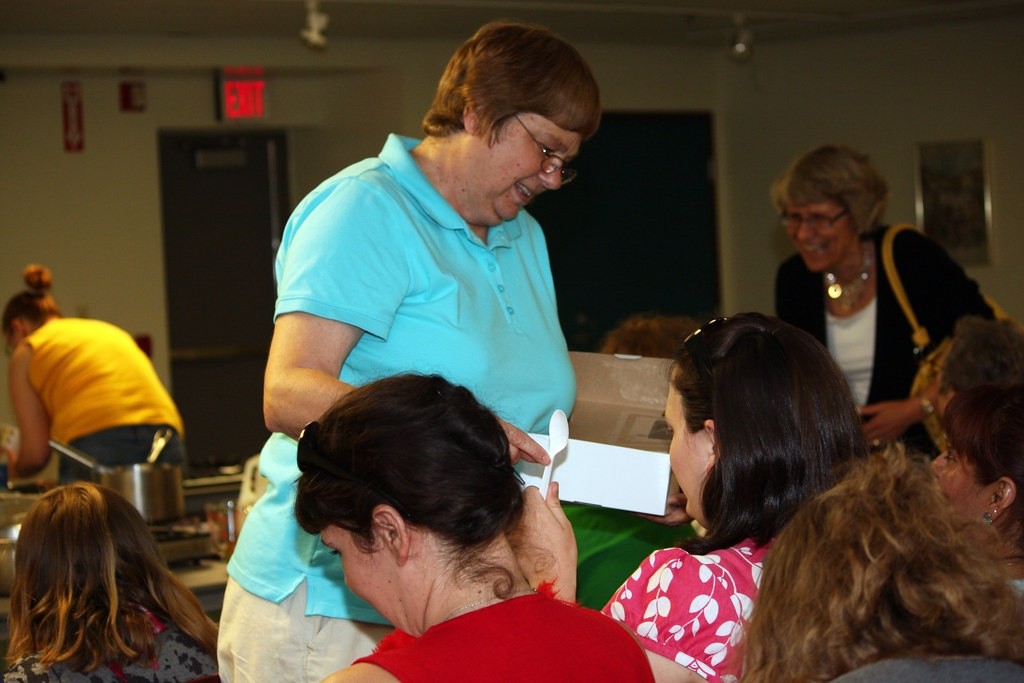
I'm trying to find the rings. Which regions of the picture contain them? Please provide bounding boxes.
[873,439,880,446]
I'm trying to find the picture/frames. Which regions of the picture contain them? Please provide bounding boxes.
[912,136,992,267]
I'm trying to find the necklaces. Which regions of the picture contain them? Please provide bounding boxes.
[826,242,869,315]
[1004,562,1023,568]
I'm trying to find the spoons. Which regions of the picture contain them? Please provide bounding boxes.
[539,409,569,501]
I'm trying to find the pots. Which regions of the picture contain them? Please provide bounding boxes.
[47,436,185,525]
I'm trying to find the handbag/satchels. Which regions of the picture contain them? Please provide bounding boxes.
[880,221,1024,454]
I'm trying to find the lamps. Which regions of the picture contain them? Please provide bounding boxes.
[298,10,331,51]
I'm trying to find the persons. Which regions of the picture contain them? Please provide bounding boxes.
[3,483,219,683]
[217,22,601,683]
[3,263,185,484]
[776,146,995,460]
[562,320,694,613]
[600,314,868,682]
[737,444,1024,683]
[292,374,654,683]
[928,319,1024,587]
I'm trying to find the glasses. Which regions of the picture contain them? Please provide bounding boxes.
[683,311,766,376]
[512,113,577,185]
[294,419,415,520]
[780,206,848,228]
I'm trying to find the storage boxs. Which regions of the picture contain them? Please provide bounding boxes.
[519,351,680,517]
